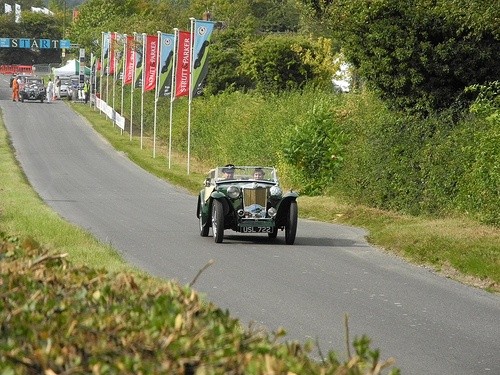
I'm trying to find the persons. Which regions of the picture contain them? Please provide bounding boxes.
[222,164,236,179]
[12,76,20,102]
[83,79,91,103]
[253,167,265,180]
[46,78,53,103]
[55,76,61,100]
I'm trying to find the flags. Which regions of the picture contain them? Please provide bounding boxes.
[91,19,216,102]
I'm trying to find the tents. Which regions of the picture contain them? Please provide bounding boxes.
[53,60,91,77]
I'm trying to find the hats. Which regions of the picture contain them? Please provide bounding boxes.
[222,164,235,173]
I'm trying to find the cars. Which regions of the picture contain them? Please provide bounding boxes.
[10,71,45,103]
[197,166,298,243]
[54,74,70,99]
[68,74,90,100]
